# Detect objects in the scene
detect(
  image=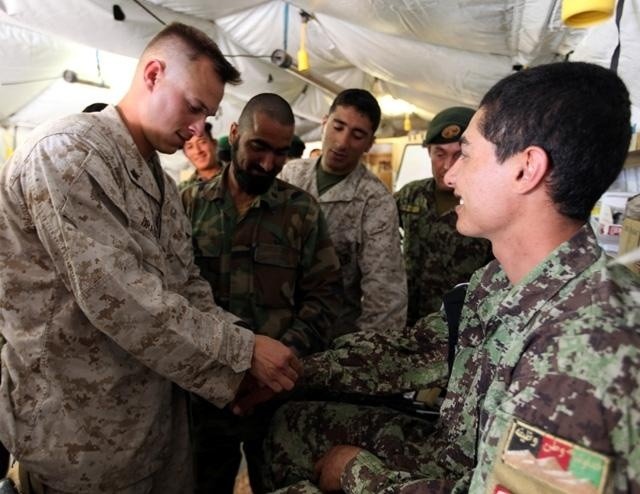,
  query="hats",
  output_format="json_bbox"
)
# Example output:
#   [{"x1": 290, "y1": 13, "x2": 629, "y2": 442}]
[
  {"x1": 424, "y1": 107, "x2": 477, "y2": 146},
  {"x1": 217, "y1": 133, "x2": 305, "y2": 160}
]
